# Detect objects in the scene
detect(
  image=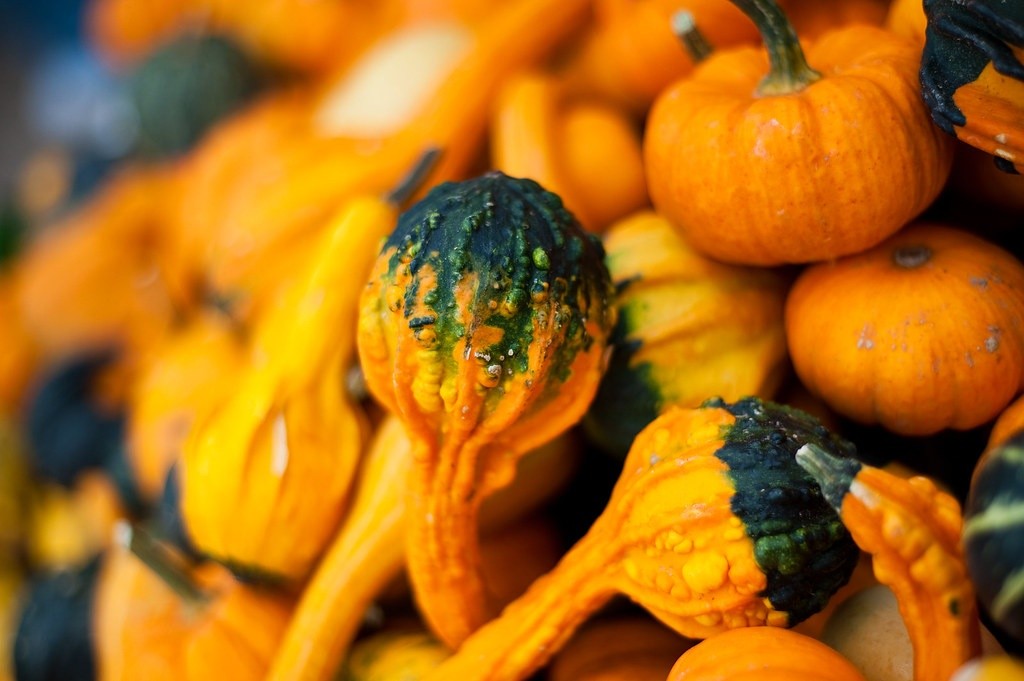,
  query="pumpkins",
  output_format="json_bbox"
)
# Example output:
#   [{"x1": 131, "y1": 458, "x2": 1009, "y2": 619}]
[{"x1": 0, "y1": 0, "x2": 1024, "y2": 681}]
